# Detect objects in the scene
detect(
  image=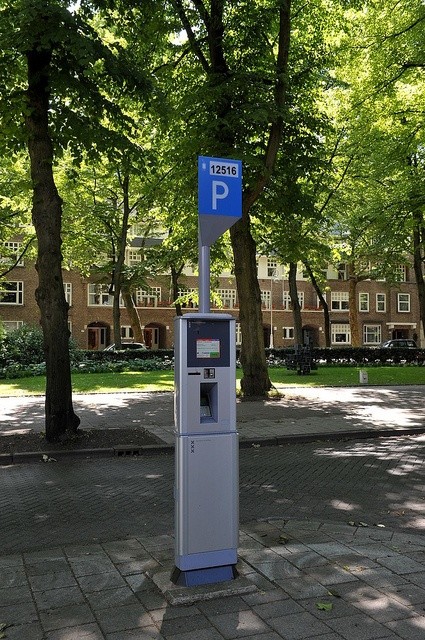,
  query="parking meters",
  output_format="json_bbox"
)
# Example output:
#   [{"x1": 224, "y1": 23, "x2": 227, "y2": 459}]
[{"x1": 173, "y1": 155, "x2": 245, "y2": 585}]
